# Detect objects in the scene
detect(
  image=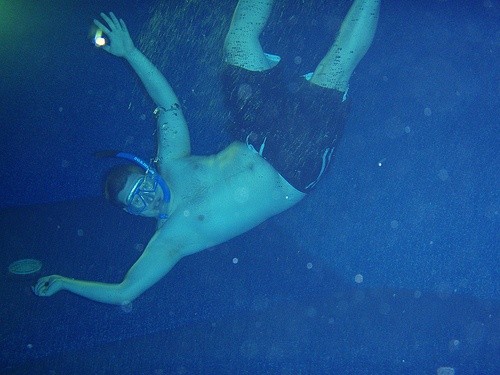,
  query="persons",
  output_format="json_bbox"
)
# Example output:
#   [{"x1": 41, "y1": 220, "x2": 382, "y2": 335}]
[{"x1": 30, "y1": 1, "x2": 382, "y2": 308}]
